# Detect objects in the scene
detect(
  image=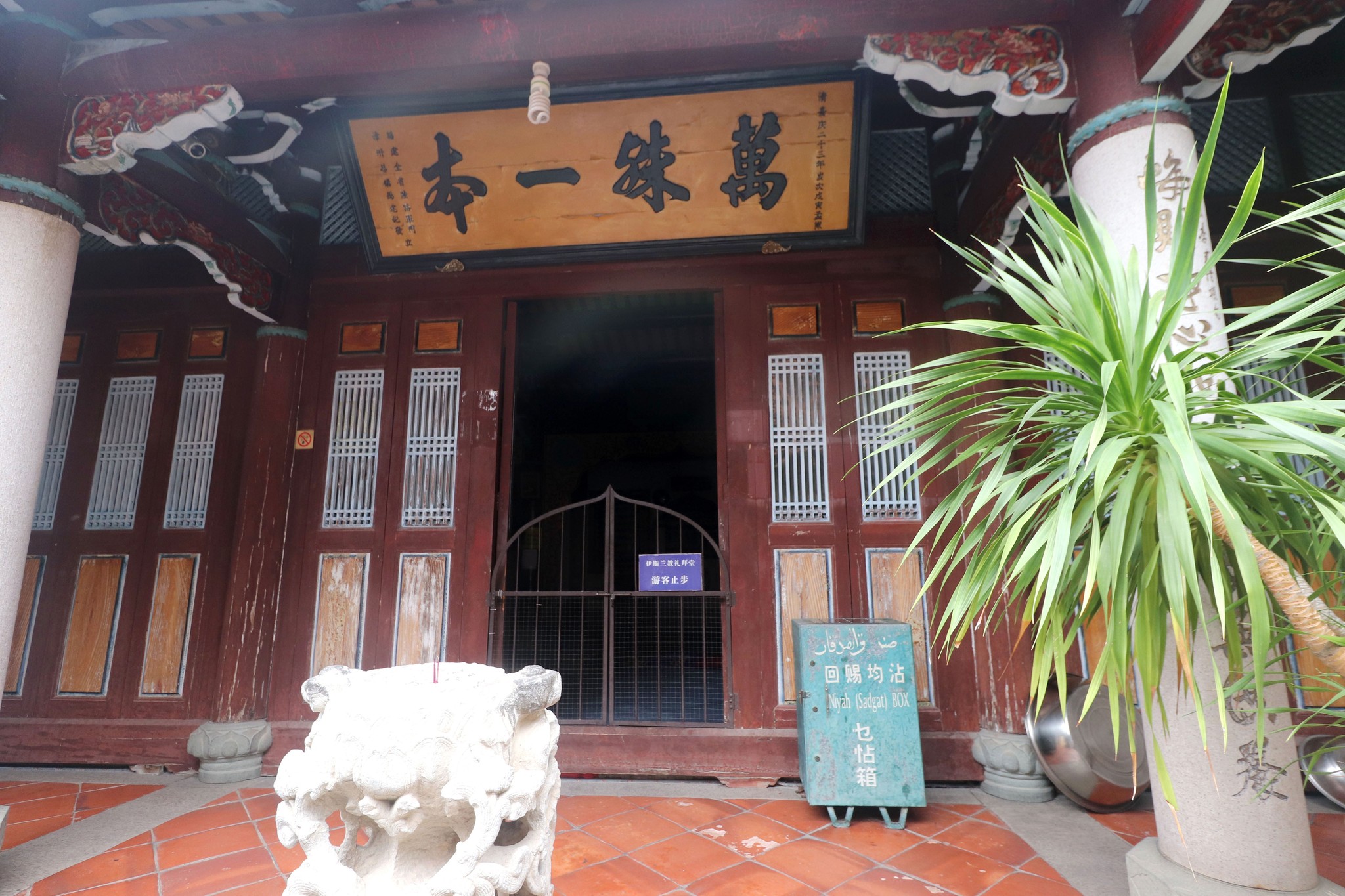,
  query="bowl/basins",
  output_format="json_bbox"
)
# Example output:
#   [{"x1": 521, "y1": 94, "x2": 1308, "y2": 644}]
[
  {"x1": 1025, "y1": 673, "x2": 1150, "y2": 813},
  {"x1": 1299, "y1": 734, "x2": 1345, "y2": 808}
]
